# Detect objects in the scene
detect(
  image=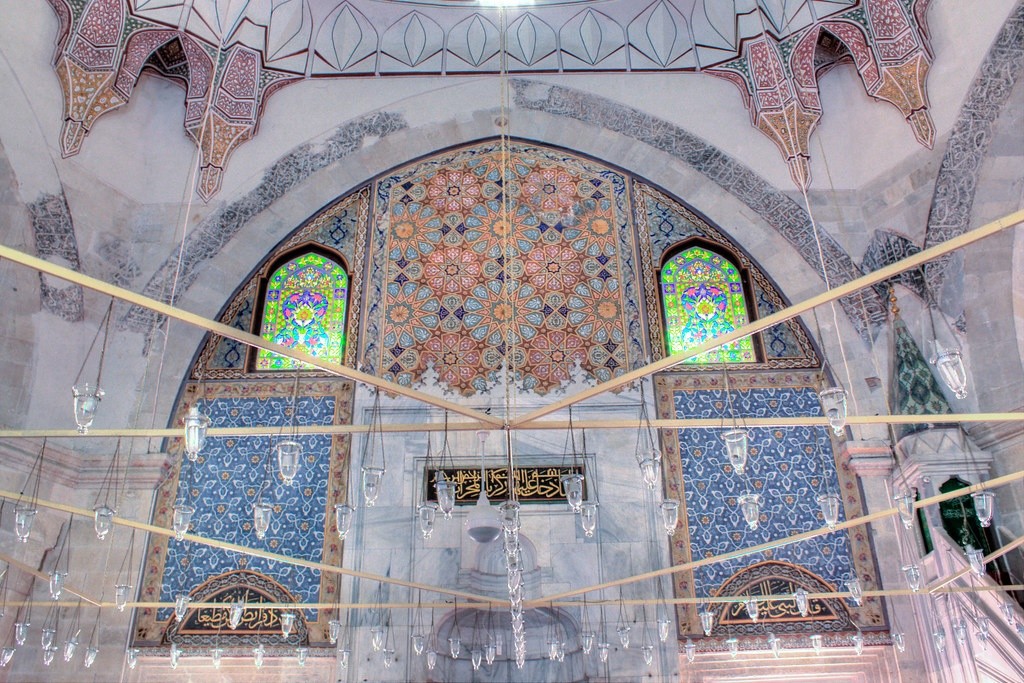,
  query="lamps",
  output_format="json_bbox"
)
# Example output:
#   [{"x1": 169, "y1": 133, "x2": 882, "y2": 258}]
[{"x1": 1, "y1": 0, "x2": 999, "y2": 680}]
